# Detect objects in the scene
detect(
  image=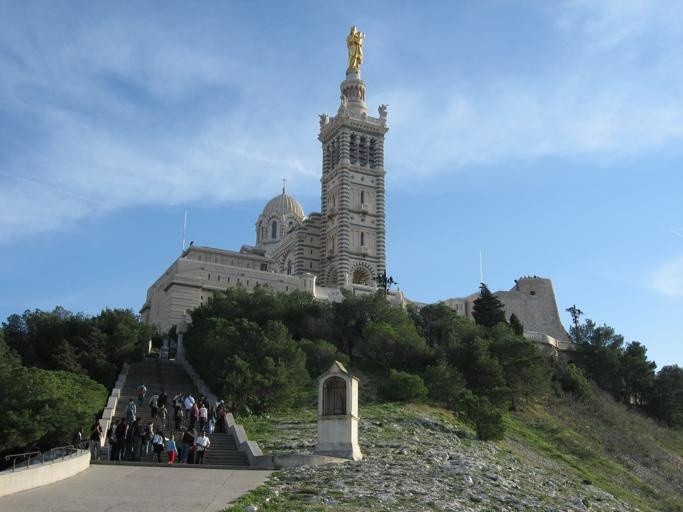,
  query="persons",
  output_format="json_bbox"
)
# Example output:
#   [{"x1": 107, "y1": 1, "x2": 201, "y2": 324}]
[
  {"x1": 90, "y1": 421, "x2": 102, "y2": 462},
  {"x1": 107, "y1": 387, "x2": 226, "y2": 464},
  {"x1": 345, "y1": 26, "x2": 362, "y2": 71},
  {"x1": 353, "y1": 31, "x2": 365, "y2": 45},
  {"x1": 72, "y1": 428, "x2": 84, "y2": 448},
  {"x1": 565, "y1": 333, "x2": 575, "y2": 344},
  {"x1": 136, "y1": 381, "x2": 147, "y2": 407}
]
